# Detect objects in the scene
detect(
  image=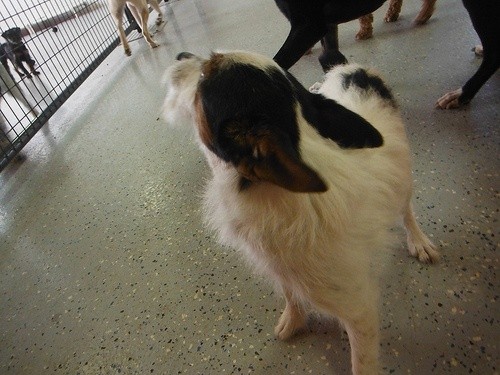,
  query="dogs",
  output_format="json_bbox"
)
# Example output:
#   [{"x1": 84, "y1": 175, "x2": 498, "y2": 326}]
[
  {"x1": 0, "y1": 26, "x2": 39, "y2": 79},
  {"x1": 108, "y1": 0, "x2": 164, "y2": 57},
  {"x1": 273, "y1": 0, "x2": 500, "y2": 110},
  {"x1": 162, "y1": 51, "x2": 441, "y2": 375}
]
